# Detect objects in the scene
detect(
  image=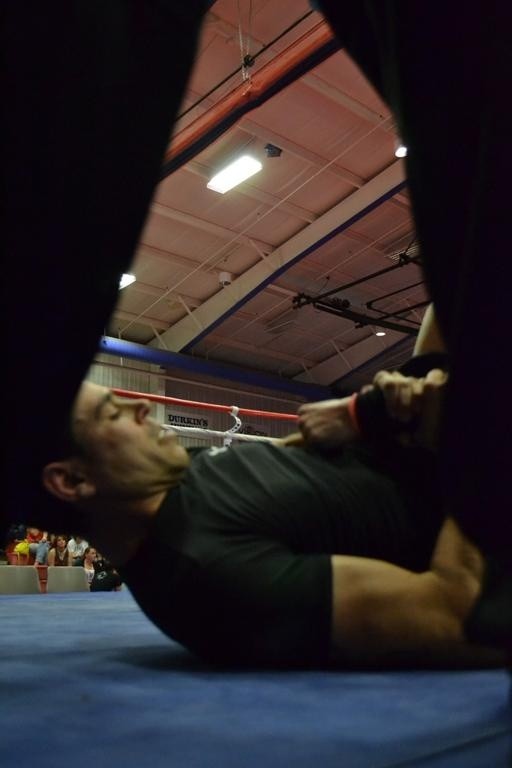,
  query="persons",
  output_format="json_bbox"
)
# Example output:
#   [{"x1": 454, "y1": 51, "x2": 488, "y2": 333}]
[
  {"x1": 1, "y1": 526, "x2": 125, "y2": 591},
  {"x1": 2, "y1": 2, "x2": 510, "y2": 599},
  {"x1": 0, "y1": 301, "x2": 510, "y2": 671}
]
